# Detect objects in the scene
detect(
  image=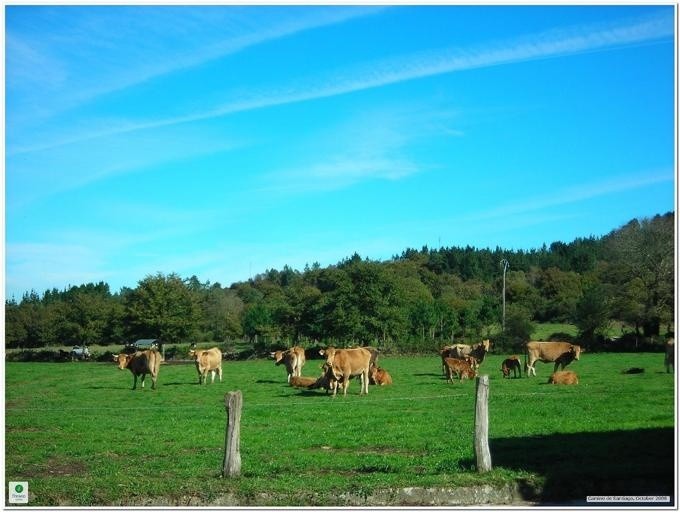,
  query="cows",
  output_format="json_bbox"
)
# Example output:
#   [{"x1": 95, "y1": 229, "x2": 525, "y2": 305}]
[
  {"x1": 440, "y1": 339, "x2": 490, "y2": 384},
  {"x1": 58, "y1": 345, "x2": 91, "y2": 362},
  {"x1": 548, "y1": 370, "x2": 579, "y2": 385},
  {"x1": 270, "y1": 346, "x2": 392, "y2": 398},
  {"x1": 188, "y1": 346, "x2": 222, "y2": 384},
  {"x1": 664, "y1": 337, "x2": 674, "y2": 374},
  {"x1": 112, "y1": 349, "x2": 161, "y2": 389},
  {"x1": 524, "y1": 340, "x2": 586, "y2": 378},
  {"x1": 500, "y1": 355, "x2": 521, "y2": 378}
]
